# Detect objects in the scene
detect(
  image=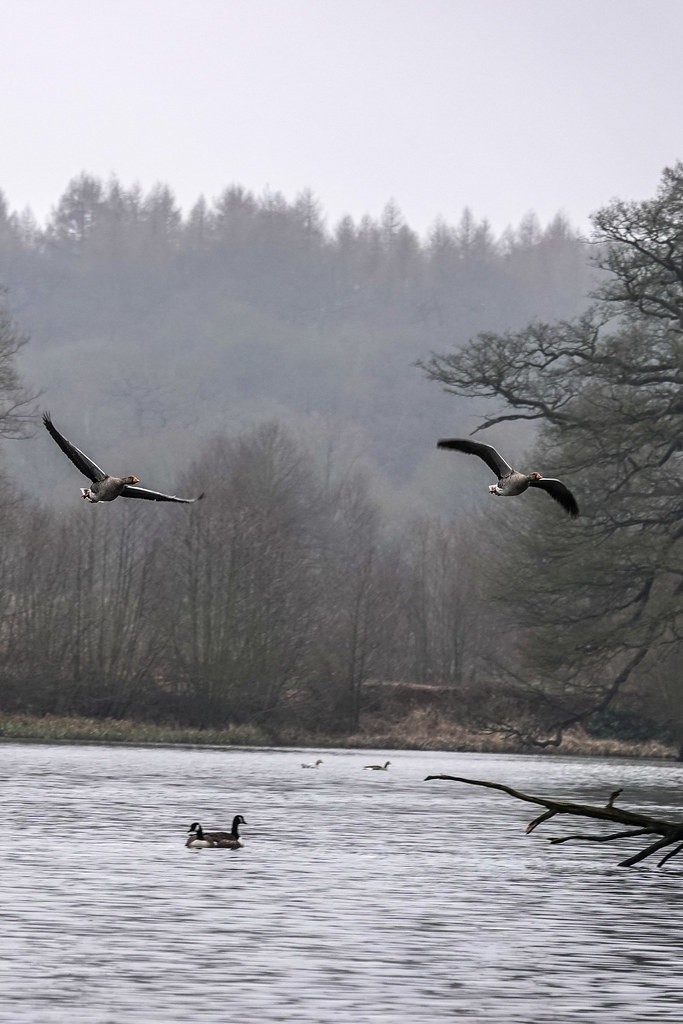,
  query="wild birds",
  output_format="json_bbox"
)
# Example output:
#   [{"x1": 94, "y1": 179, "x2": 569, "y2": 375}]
[
  {"x1": 437, "y1": 439, "x2": 580, "y2": 520},
  {"x1": 42, "y1": 410, "x2": 205, "y2": 503},
  {"x1": 301, "y1": 760, "x2": 323, "y2": 768},
  {"x1": 364, "y1": 761, "x2": 392, "y2": 771},
  {"x1": 185, "y1": 815, "x2": 248, "y2": 846},
  {"x1": 188, "y1": 823, "x2": 240, "y2": 848}
]
